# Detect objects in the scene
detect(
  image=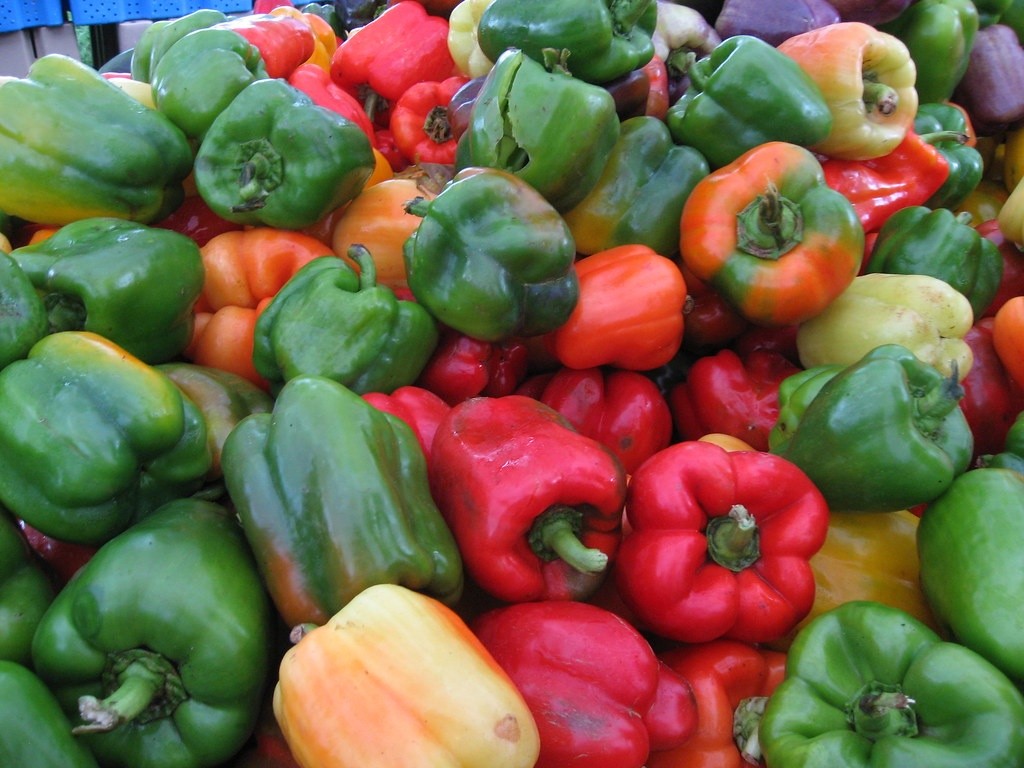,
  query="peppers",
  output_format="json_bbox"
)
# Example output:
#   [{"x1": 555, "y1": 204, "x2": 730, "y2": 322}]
[{"x1": 0, "y1": 0, "x2": 1024, "y2": 768}]
